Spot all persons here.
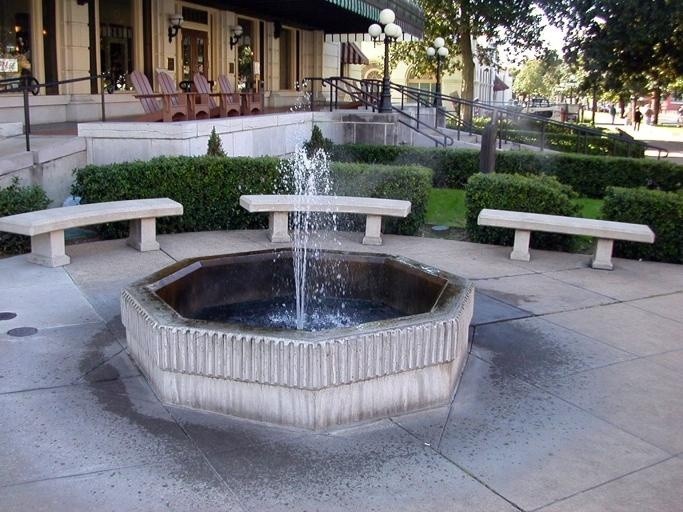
[633,105,643,131]
[610,104,616,124]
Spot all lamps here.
[165,12,186,44]
[228,24,245,50]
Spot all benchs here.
[0,196,188,270]
[475,205,658,272]
[236,192,414,247]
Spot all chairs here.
[337,79,383,110]
[124,67,265,123]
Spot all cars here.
[564,93,682,128]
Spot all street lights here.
[368,9,404,113]
[426,36,448,105]
